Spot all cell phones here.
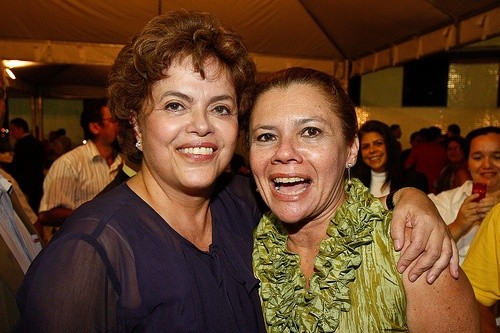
[471,181,487,204]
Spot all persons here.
[247,66,481,333]
[37,95,125,225]
[92,117,143,199]
[429,126,500,270]
[461,200,500,332]
[15,7,460,333]
[0,98,475,249]
